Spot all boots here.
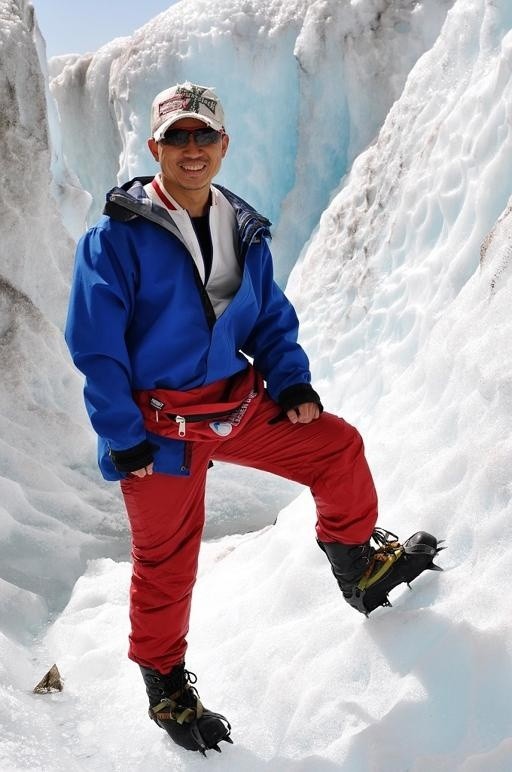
[140,663,233,754]
[315,530,441,615]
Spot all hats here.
[161,128,221,146]
[151,83,225,142]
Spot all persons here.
[62,78,437,756]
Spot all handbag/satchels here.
[141,365,264,442]
